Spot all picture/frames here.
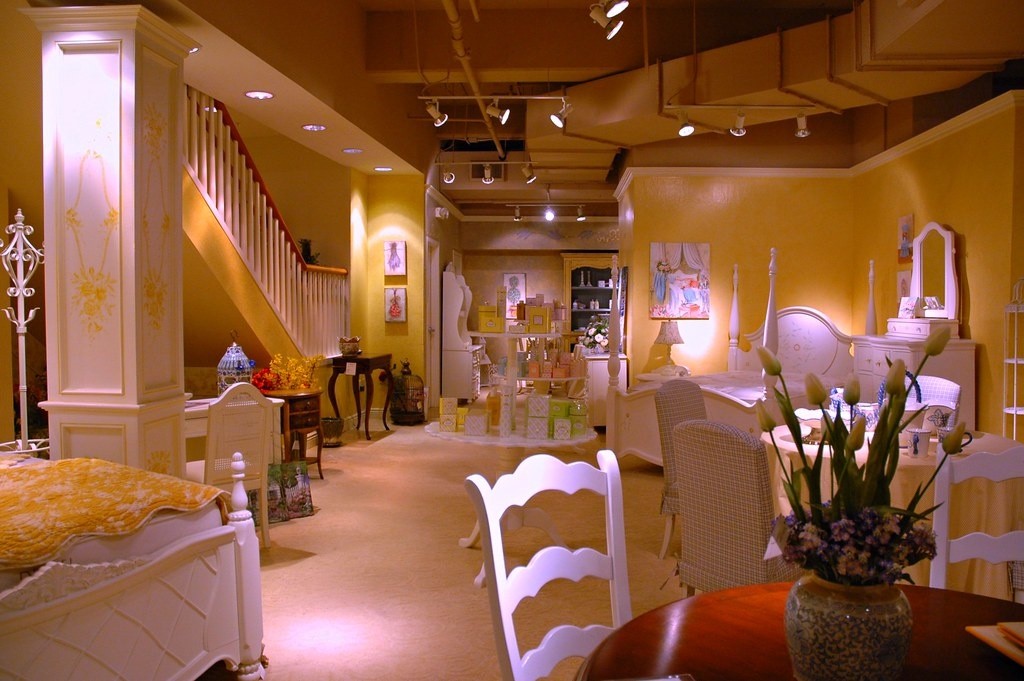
[383,241,407,276]
[384,287,407,322]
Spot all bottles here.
[590,299,595,310]
[573,300,578,309]
[517,300,525,319]
[595,299,599,309]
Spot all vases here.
[786,573,914,681]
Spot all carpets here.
[425,407,598,447]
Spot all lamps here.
[676,111,694,137]
[730,108,747,137]
[590,0,629,40]
[425,97,586,223]
[651,319,691,377]
[795,110,811,137]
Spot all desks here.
[574,582,1024,681]
[761,426,1024,601]
[465,330,563,431]
[329,354,395,441]
[185,397,285,466]
[634,372,689,382]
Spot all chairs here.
[465,375,1024,681]
[185,381,274,549]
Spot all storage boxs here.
[439,284,591,441]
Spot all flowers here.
[756,328,973,587]
[249,349,324,392]
[582,316,609,351]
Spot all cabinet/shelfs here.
[561,253,628,428]
[260,388,325,480]
[1002,278,1023,440]
[442,262,483,405]
[852,317,974,431]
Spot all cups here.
[598,280,605,288]
[936,427,973,448]
[830,392,883,430]
[907,428,932,459]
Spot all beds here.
[0,451,266,681]
[605,246,879,468]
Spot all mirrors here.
[909,222,960,319]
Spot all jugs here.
[897,402,929,448]
[922,399,956,436]
